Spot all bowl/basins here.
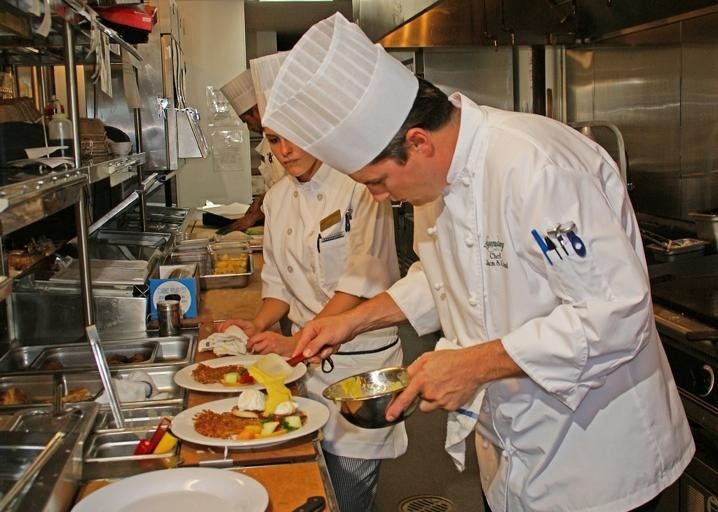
[109,142,132,154]
[322,365,420,429]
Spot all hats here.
[249,50,291,129]
[219,71,259,117]
[264,13,420,176]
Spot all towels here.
[436,338,485,472]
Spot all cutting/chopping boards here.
[150,253,283,329]
[74,461,331,512]
[179,322,318,466]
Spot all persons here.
[215,49,409,512]
[261,11,697,512]
[220,68,286,231]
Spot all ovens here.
[566,4,718,512]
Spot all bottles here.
[47,103,74,159]
[158,300,181,338]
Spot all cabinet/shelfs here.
[1,1,147,340]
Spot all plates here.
[116,205,193,241]
[29,340,159,372]
[164,238,253,253]
[173,355,307,392]
[0,334,194,373]
[71,467,269,512]
[85,430,178,462]
[0,375,105,407]
[170,396,330,449]
[91,228,172,257]
[148,252,254,289]
[93,406,183,433]
[248,235,264,250]
